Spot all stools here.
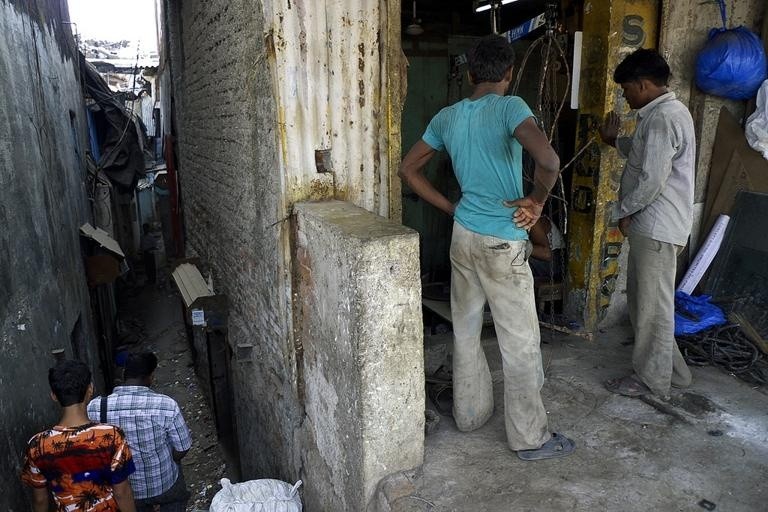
[532,275,562,313]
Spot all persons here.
[597,48,696,400]
[527,211,566,277]
[20,359,139,511]
[399,34,574,461]
[87,349,192,511]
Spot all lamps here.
[472,0,519,13]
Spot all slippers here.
[604,375,651,398]
[516,433,575,461]
